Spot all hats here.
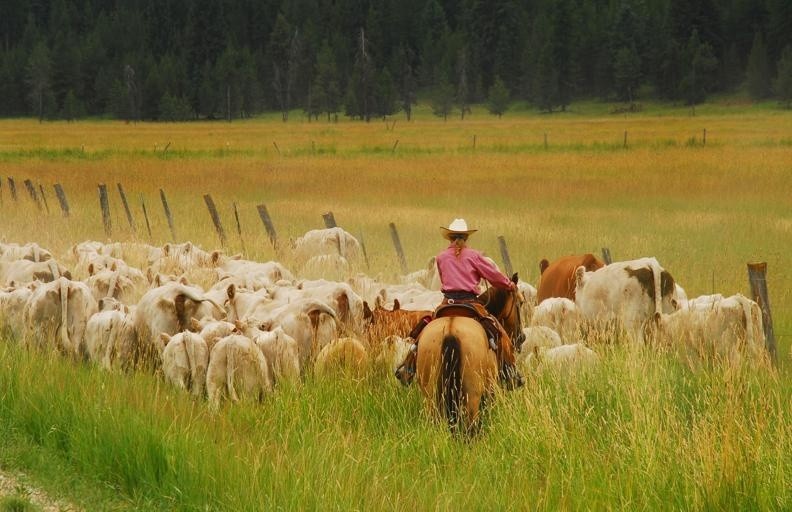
[440,218,478,240]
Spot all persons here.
[395,218,527,392]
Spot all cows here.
[0,227,445,417]
[479,253,765,379]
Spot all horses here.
[414,272,526,444]
